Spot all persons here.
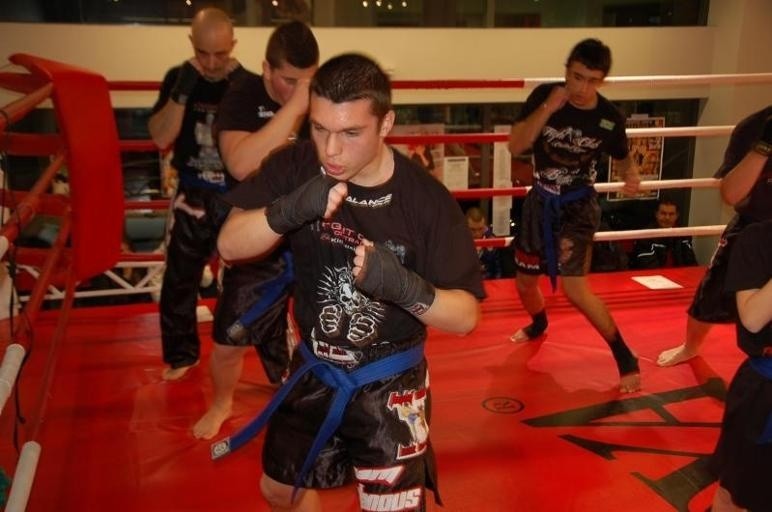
[190,14,322,441]
[412,146,436,170]
[630,195,699,271]
[211,52,490,512]
[147,6,291,391]
[112,240,143,291]
[465,204,502,279]
[703,217,770,511]
[507,35,642,396]
[654,105,772,367]
[589,214,623,273]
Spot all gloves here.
[353,242,437,318]
[752,115,771,157]
[265,174,340,237]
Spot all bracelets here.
[540,100,554,117]
